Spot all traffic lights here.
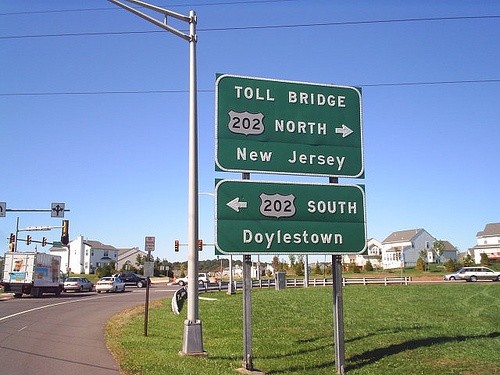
[197,240,202,251]
[175,240,179,252]
[42,237,46,246]
[9,233,15,243]
[61,219,70,239]
[27,234,31,245]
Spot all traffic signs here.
[51,202,64,217]
[214,73,365,179]
[0,202,6,217]
[214,178,368,257]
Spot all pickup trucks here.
[176,272,221,286]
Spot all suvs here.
[114,273,150,288]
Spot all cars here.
[63,277,93,293]
[96,277,125,293]
[460,268,500,282]
[444,267,466,280]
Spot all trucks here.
[2,251,64,297]
